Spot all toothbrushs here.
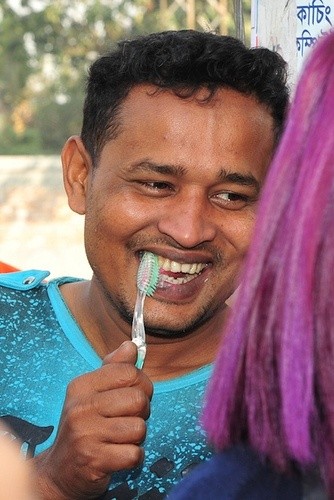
[131,252,160,370]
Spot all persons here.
[0,30,288,500]
[166,29,334,500]
